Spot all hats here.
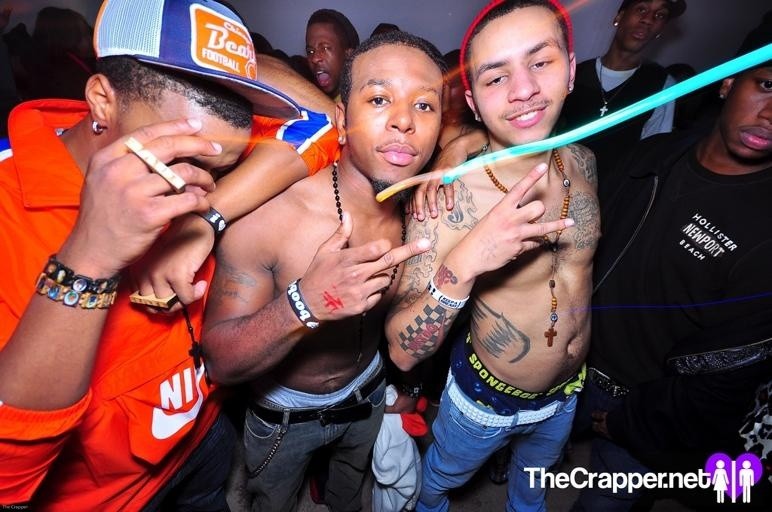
[618,0,686,21]
[91,0,301,120]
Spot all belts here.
[445,366,567,427]
[250,366,386,426]
